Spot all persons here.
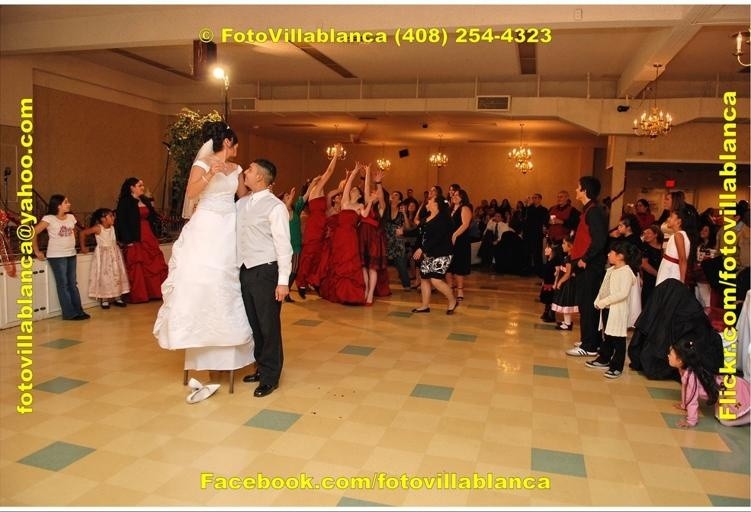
[153,121,257,374]
[31,193,91,321]
[233,157,293,397]
[80,208,131,310]
[115,177,168,304]
[277,140,751,430]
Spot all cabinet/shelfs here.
[0,260,39,329]
[39,254,101,322]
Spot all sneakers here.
[540,311,623,378]
[113,301,127,307]
[73,313,91,320]
[101,300,110,309]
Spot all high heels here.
[186,377,222,404]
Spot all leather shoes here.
[242,369,262,382]
[283,274,464,315]
[254,382,279,397]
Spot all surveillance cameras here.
[617,105,629,112]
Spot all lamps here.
[633,64,672,137]
[428,133,448,169]
[326,123,347,161]
[506,123,531,162]
[736,27,751,70]
[376,143,391,172]
[516,163,534,175]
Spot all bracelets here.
[202,175,210,184]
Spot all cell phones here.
[550,215,556,224]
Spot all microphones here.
[5,166,11,175]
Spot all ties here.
[495,224,498,239]
